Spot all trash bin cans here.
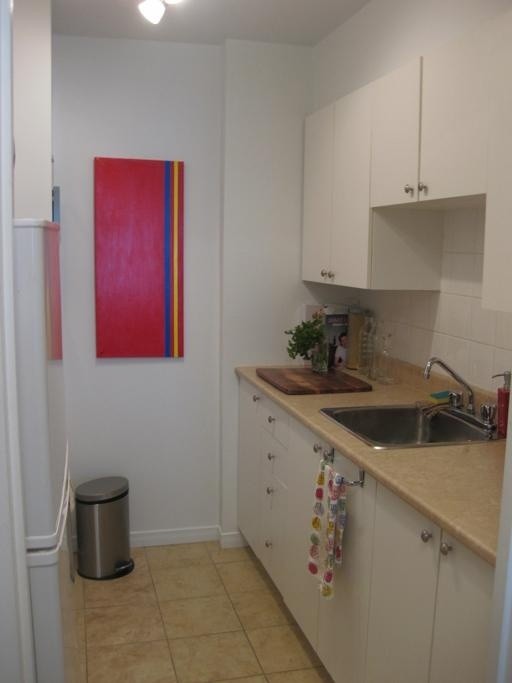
[74,474,133,581]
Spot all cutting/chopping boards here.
[256,367,372,394]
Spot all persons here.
[334,332,348,367]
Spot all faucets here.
[423,357,475,414]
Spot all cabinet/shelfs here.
[479,10,510,304]
[371,31,485,212]
[237,378,290,598]
[365,437,498,682]
[302,86,443,293]
[282,418,376,680]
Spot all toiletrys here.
[490,371,512,438]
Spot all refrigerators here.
[13,219,73,683]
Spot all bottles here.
[356,316,399,384]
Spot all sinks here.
[318,402,506,450]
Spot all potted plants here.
[285,312,329,374]
